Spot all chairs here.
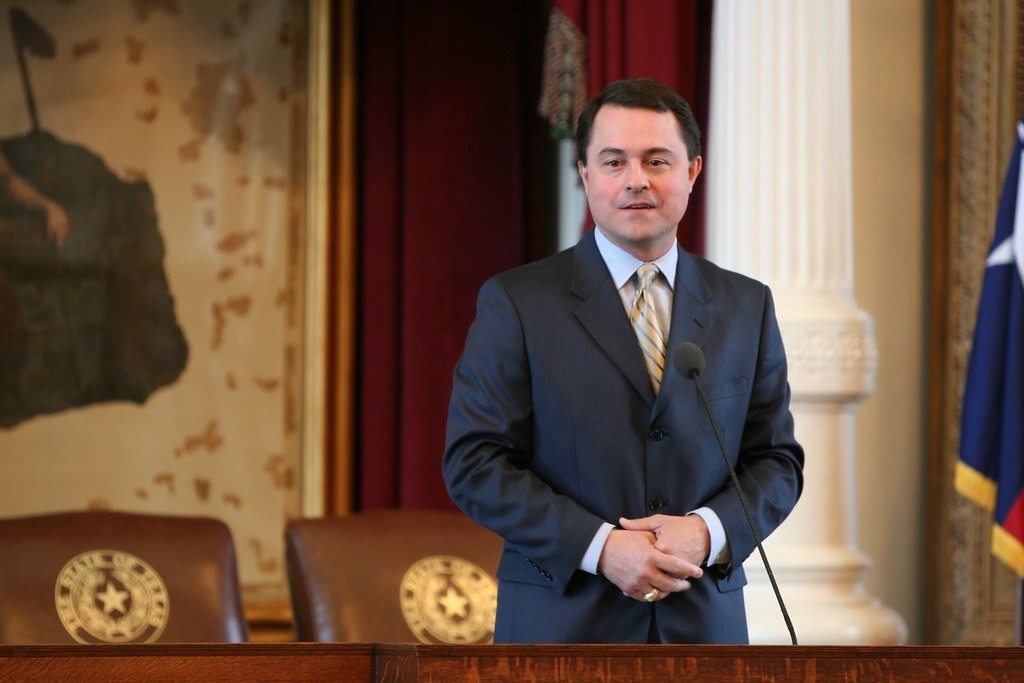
[0,509,248,645]
[283,512,505,645]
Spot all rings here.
[643,589,658,603]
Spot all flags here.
[954,116,1024,578]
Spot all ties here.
[631,264,668,395]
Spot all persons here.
[443,78,804,644]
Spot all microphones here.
[673,342,801,645]
